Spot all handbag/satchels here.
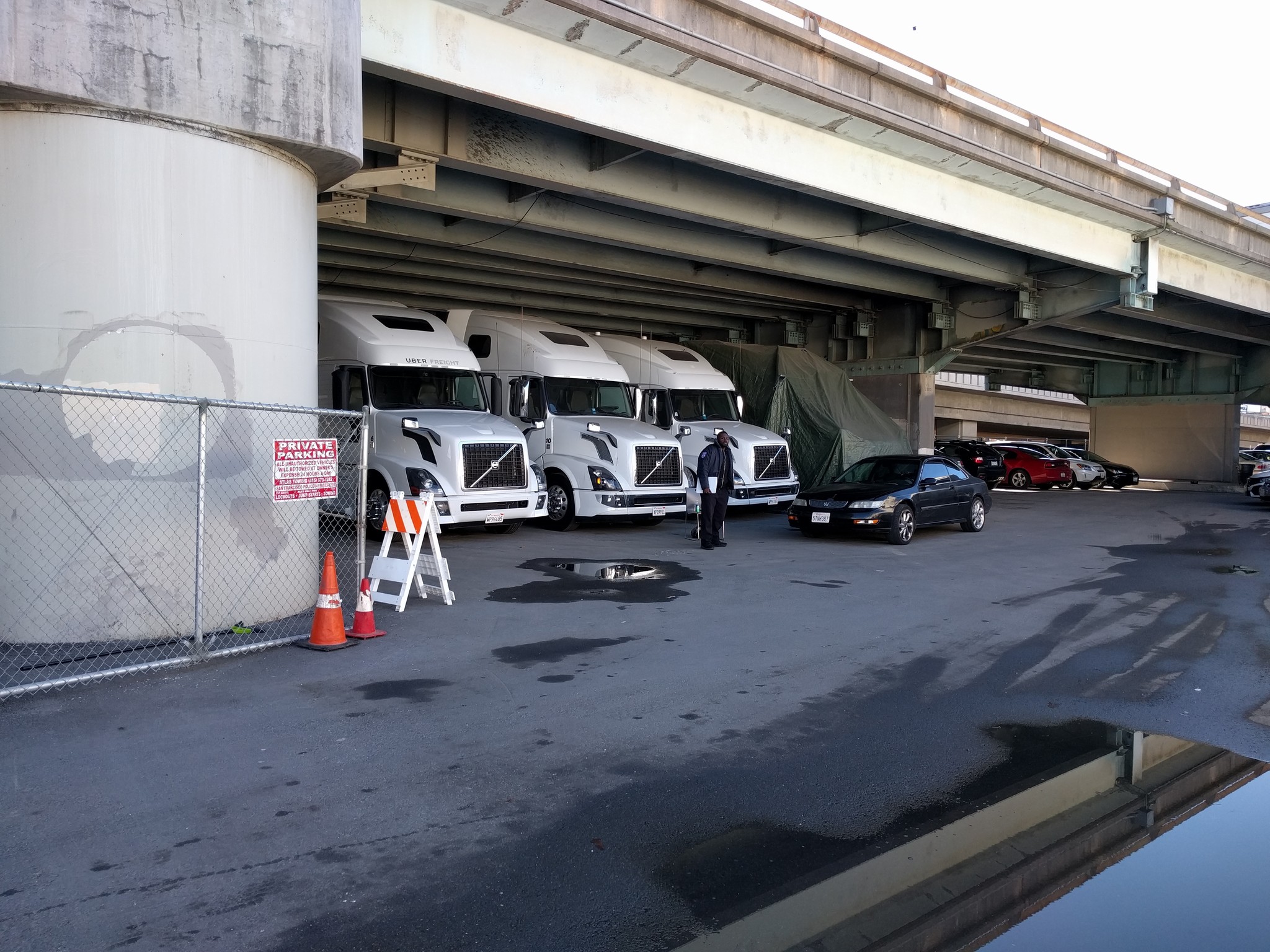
[691,523,701,538]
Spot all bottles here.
[693,500,699,511]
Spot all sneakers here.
[712,540,727,547]
[701,543,714,549]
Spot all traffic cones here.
[346,578,388,641]
[294,551,362,652]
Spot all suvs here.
[934,439,1008,491]
[1253,443,1270,451]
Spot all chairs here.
[677,399,698,418]
[418,382,439,405]
[567,391,591,412]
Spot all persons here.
[697,431,734,549]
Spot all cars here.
[1060,447,1140,489]
[985,441,1107,491]
[987,446,1073,489]
[1244,470,1270,502]
[1238,452,1270,474]
[932,448,965,471]
[1239,450,1270,461]
[786,455,996,544]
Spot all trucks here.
[583,331,802,513]
[318,296,550,536]
[433,310,691,531]
